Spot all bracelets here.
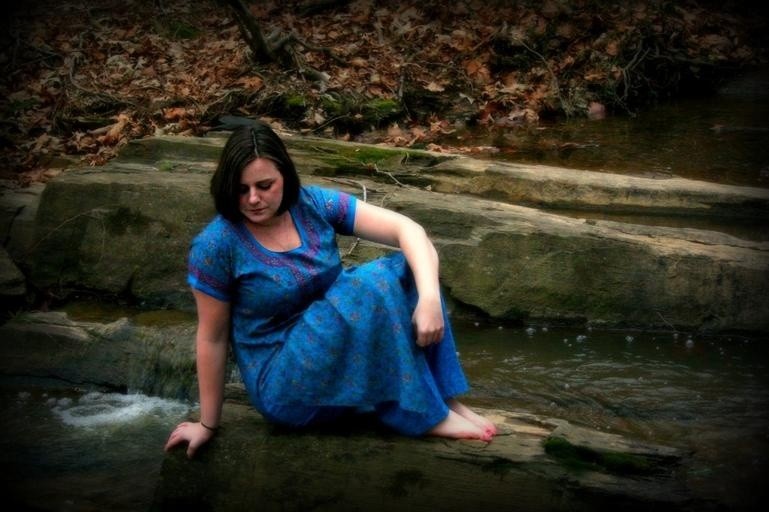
[201,420,220,431]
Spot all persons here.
[165,124,497,459]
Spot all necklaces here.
[262,215,288,253]
